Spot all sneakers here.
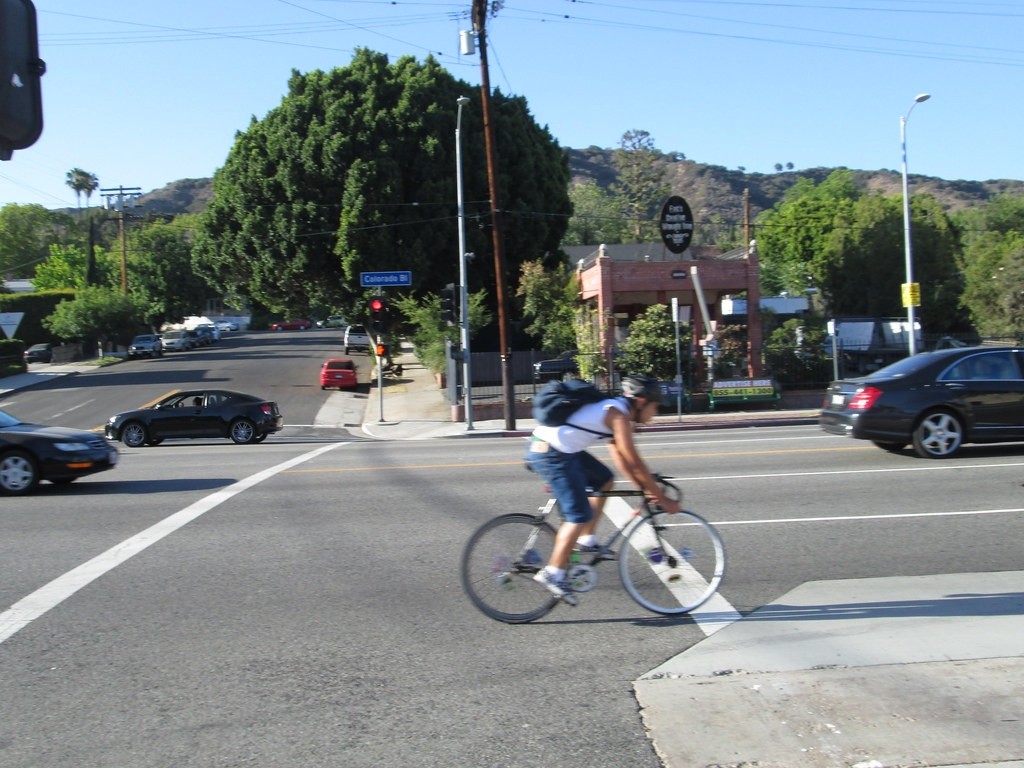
[573,542,619,561]
[533,568,578,605]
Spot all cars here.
[0,411,119,498]
[105,389,282,448]
[320,359,359,392]
[129,316,239,358]
[23,344,54,364]
[817,346,1024,460]
[535,349,579,381]
[271,319,311,331]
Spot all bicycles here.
[460,472,726,625]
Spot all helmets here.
[623,371,664,402]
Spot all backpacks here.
[532,378,630,438]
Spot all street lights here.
[455,93,478,436]
[898,93,933,357]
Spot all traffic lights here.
[376,343,390,356]
[368,295,387,315]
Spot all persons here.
[523,373,680,607]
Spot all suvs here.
[344,324,370,354]
[317,315,349,328]
